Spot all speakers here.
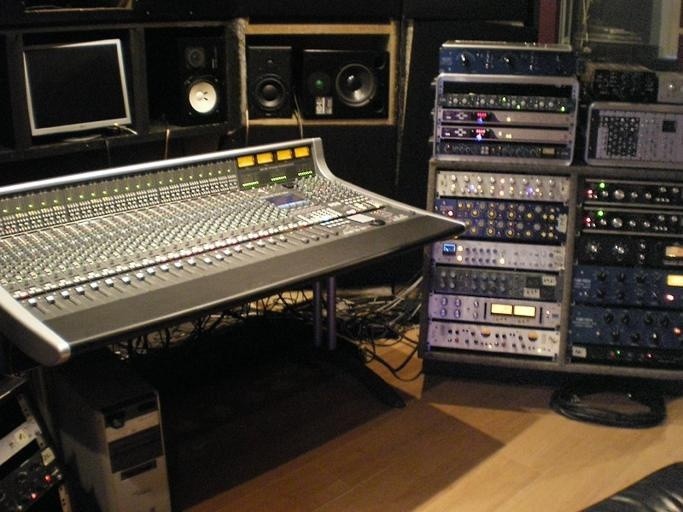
[148,26,228,127]
[302,49,389,119]
[246,45,293,119]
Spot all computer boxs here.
[44,347,172,512]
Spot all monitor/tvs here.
[22,38,133,142]
